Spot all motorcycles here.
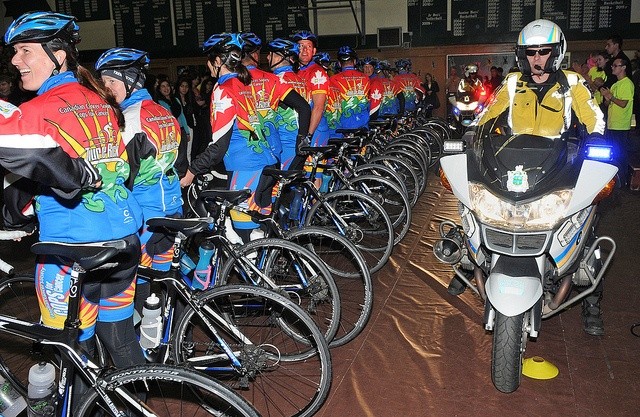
[448,89,486,134]
[433,74,619,392]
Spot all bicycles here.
[297,144,412,253]
[180,188,340,361]
[307,137,411,252]
[0,238,263,416]
[180,168,373,348]
[0,216,332,416]
[335,126,419,219]
[279,144,394,277]
[369,102,452,169]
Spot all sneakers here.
[581,296,604,336]
[448,265,475,296]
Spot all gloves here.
[296,136,309,156]
[79,158,102,187]
[461,126,480,147]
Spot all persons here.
[0,77,13,102]
[483,76,491,92]
[192,74,214,155]
[267,38,308,171]
[143,74,159,103]
[95,46,184,339]
[490,66,503,88]
[8,73,38,107]
[600,57,634,189]
[594,37,632,118]
[294,30,330,205]
[448,66,460,93]
[154,80,191,179]
[361,57,383,120]
[586,59,592,69]
[379,58,426,116]
[477,76,482,83]
[240,33,311,169]
[589,50,599,66]
[448,19,606,335]
[422,73,440,117]
[498,67,505,79]
[0,11,144,417]
[456,63,483,102]
[585,52,611,104]
[157,73,169,84]
[326,46,371,164]
[179,32,277,241]
[174,78,197,167]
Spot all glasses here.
[612,63,624,68]
[526,48,553,56]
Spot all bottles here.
[27,362,59,417]
[319,169,333,193]
[245,228,265,275]
[139,292,163,350]
[289,182,304,219]
[192,239,215,291]
[350,156,357,167]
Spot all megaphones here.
[433,228,464,264]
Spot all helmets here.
[515,19,567,76]
[337,45,358,60]
[395,59,410,67]
[4,11,81,47]
[375,60,391,70]
[292,31,318,48]
[241,33,262,46]
[361,57,377,66]
[200,32,247,52]
[464,65,479,78]
[265,38,300,56]
[95,48,151,72]
[312,52,331,67]
[177,67,189,76]
